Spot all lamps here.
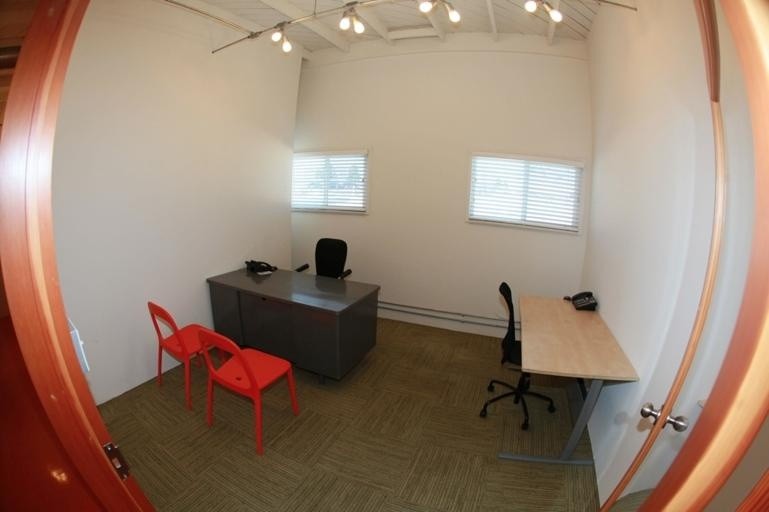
[210,0,638,55]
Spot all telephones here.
[569,291,597,311]
[246,260,277,272]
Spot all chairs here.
[148,302,225,412]
[294,237,352,280]
[480,281,556,431]
[199,329,300,455]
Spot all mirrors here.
[1,0,768,510]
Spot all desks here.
[207,265,381,385]
[498,294,639,465]
